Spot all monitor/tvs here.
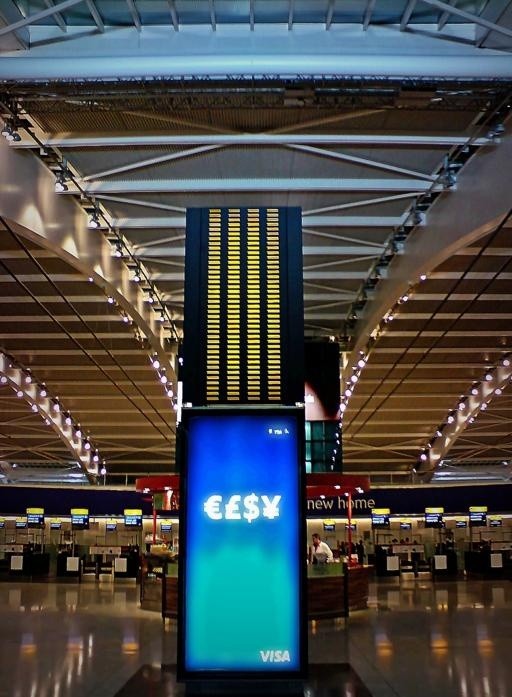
[372,515,390,529]
[425,514,442,528]
[16,522,26,529]
[71,514,90,530]
[324,525,335,532]
[456,522,466,528]
[106,524,117,532]
[469,513,486,527]
[0,522,5,528]
[26,514,44,528]
[489,520,501,527]
[400,523,411,530]
[50,523,61,530]
[345,525,356,531]
[161,525,172,532]
[124,516,143,528]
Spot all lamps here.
[2,125,10,137]
[88,243,180,412]
[6,131,15,141]
[1,363,106,481]
[421,356,511,466]
[55,177,65,192]
[88,215,99,229]
[379,123,507,268]
[330,273,427,469]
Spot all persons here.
[388,537,420,565]
[336,539,375,565]
[309,532,334,563]
